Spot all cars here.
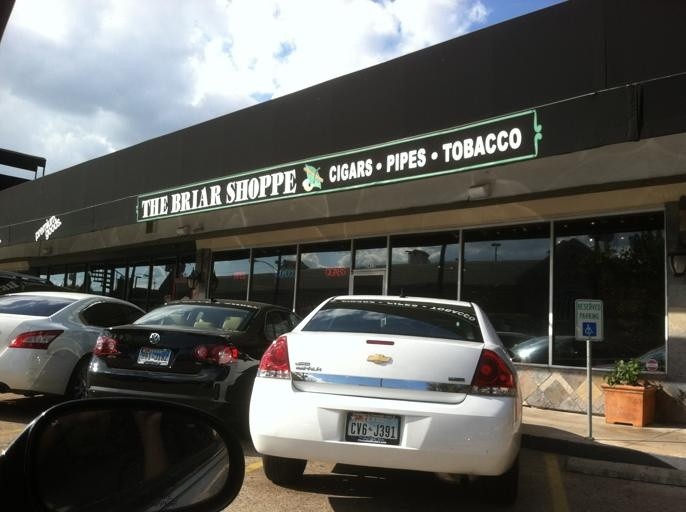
[497,331,668,372]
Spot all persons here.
[38,408,169,511]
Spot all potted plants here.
[602,360,659,425]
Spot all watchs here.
[51,419,65,440]
